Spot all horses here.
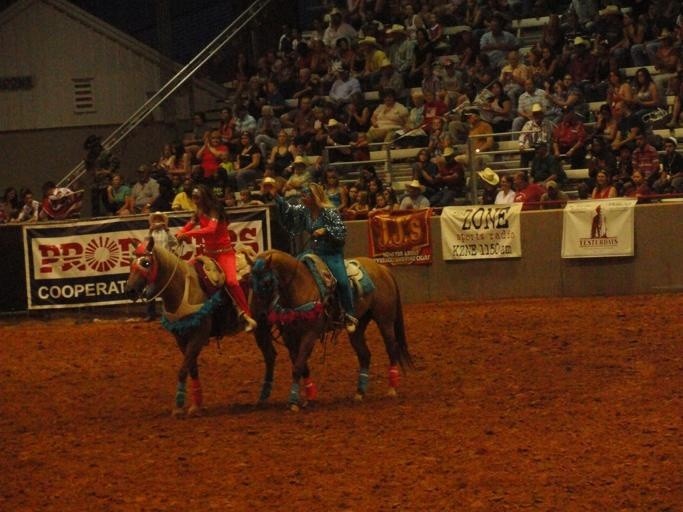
[124,237,277,420]
[240,249,416,413]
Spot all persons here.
[174,184,258,333]
[262,183,357,334]
[83,136,118,217]
[0,181,75,224]
[106,0,683,225]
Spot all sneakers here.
[245,321,254,332]
[345,317,356,333]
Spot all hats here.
[329,7,405,72]
[599,4,619,15]
[573,36,591,49]
[658,28,672,40]
[545,180,558,190]
[463,106,479,114]
[532,104,547,114]
[405,148,500,193]
[258,118,339,191]
[666,136,678,147]
